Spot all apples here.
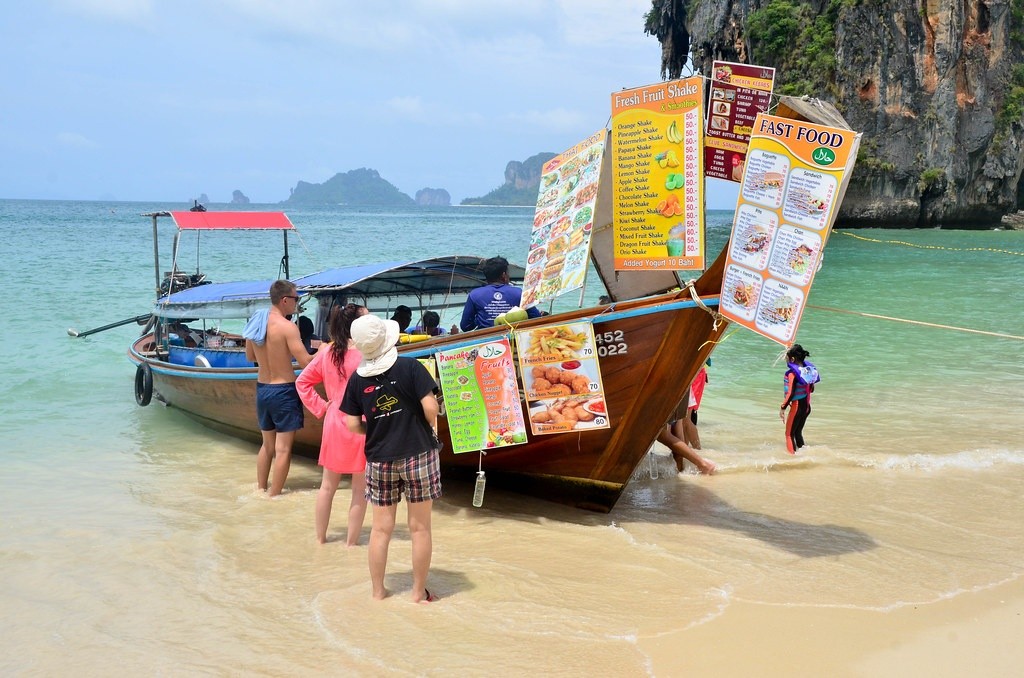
[513,431,526,442]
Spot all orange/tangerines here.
[665,173,684,189]
[656,193,683,217]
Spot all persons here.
[681,349,713,451]
[254,314,292,367]
[245,280,328,496]
[388,305,413,335]
[658,387,716,475]
[460,255,541,334]
[293,316,321,359]
[338,313,439,603]
[294,303,377,548]
[779,343,821,455]
[410,310,445,338]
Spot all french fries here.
[527,325,589,362]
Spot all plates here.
[530,405,607,430]
[582,399,606,417]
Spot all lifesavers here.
[135,362,153,406]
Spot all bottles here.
[472,471,486,508]
[649,450,658,480]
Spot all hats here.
[351,315,400,377]
[390,304,412,321]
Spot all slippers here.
[424,588,433,601]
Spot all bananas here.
[666,120,684,143]
[488,429,499,442]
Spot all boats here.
[65,93,854,516]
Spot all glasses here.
[396,316,412,322]
[280,295,299,302]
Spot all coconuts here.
[494,305,528,325]
